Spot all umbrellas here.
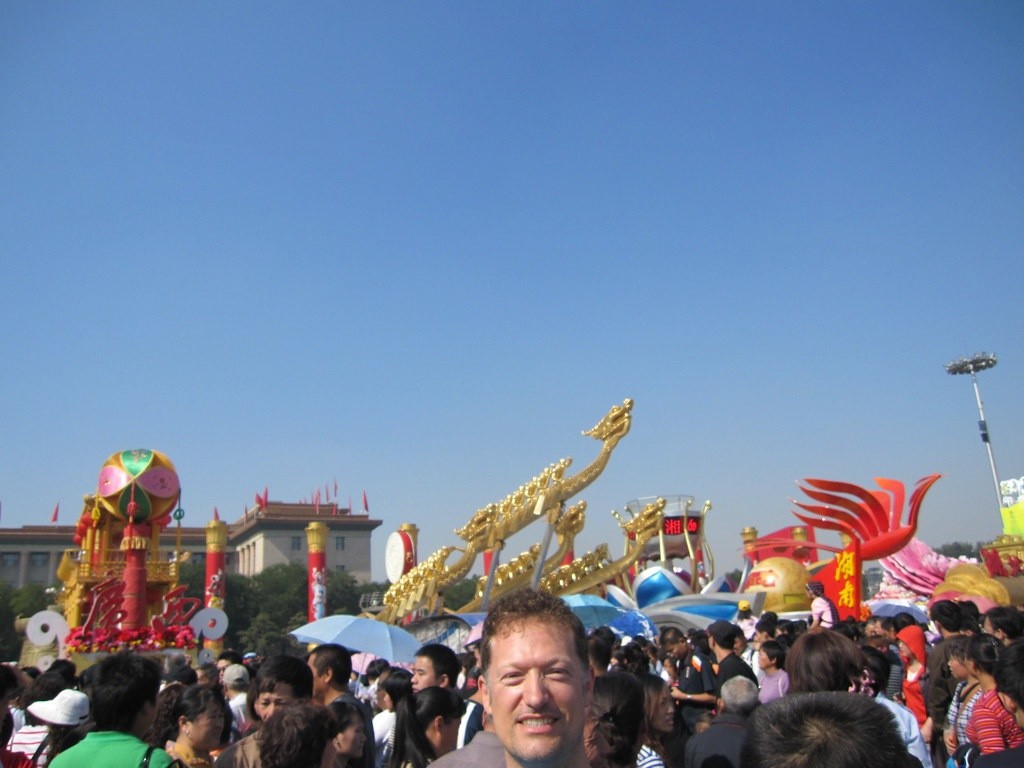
[561,594,628,628]
[289,615,424,696]
[607,611,657,637]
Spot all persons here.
[478,587,595,768]
[0,642,506,768]
[584,582,1024,768]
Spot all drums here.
[385,531,415,584]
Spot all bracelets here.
[687,695,691,702]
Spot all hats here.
[222,663,250,686]
[26,687,90,726]
[737,599,750,611]
[162,666,198,686]
[706,620,737,644]
